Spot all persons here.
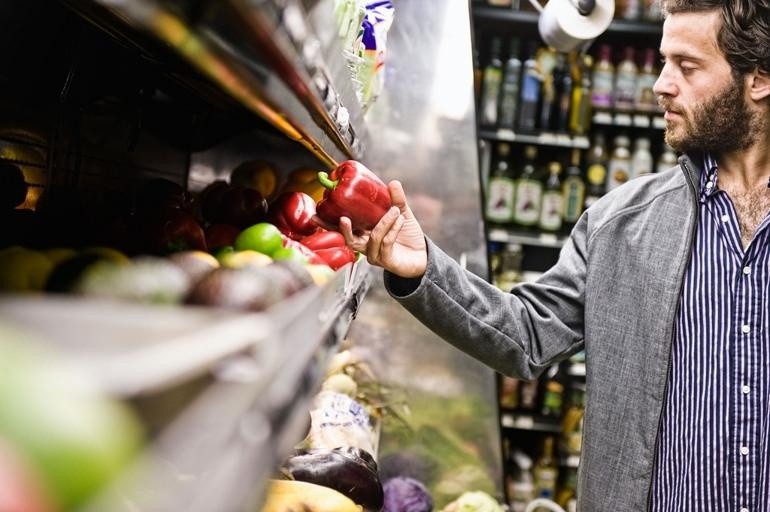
[333,1,770,512]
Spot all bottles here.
[487,239,558,293]
[471,30,663,136]
[502,434,578,512]
[498,357,585,425]
[485,133,679,236]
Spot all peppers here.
[138,159,394,272]
